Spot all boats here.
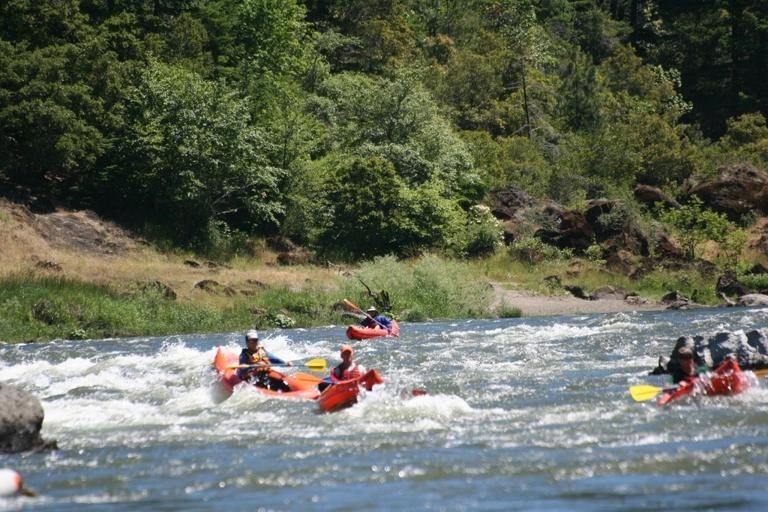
[345,317,399,340]
[658,371,758,407]
[212,348,429,415]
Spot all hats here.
[341,345,353,355]
[246,332,258,341]
[367,306,378,314]
[678,347,692,355]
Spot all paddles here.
[628,377,697,401]
[343,299,399,337]
[294,373,426,396]
[225,357,327,372]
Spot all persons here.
[673,348,705,383]
[359,306,392,332]
[318,346,367,392]
[239,332,293,391]
